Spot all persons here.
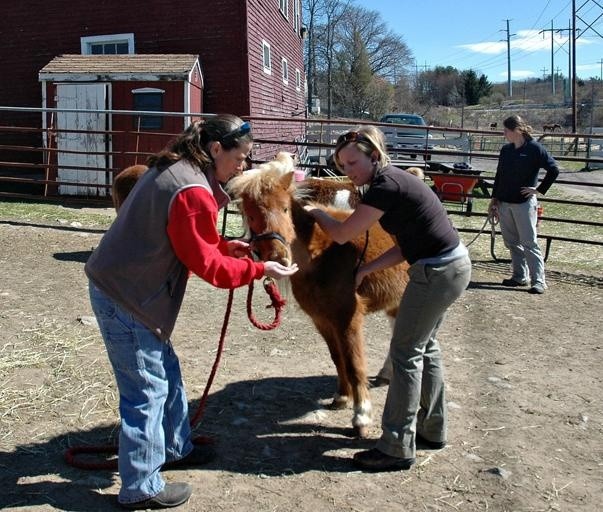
[82,112,300,510]
[302,125,472,471]
[487,113,560,294]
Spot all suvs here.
[376,115,433,161]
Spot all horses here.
[268,151,425,211]
[543,124,561,133]
[112,165,150,216]
[491,123,497,130]
[223,164,416,439]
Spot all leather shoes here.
[125,482,191,509]
[158,442,211,469]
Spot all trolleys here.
[420,169,482,218]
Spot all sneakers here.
[530,283,544,294]
[354,449,417,472]
[416,433,443,449]
[503,278,528,286]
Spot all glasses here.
[217,122,250,141]
[335,132,370,147]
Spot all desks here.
[424,158,496,200]
